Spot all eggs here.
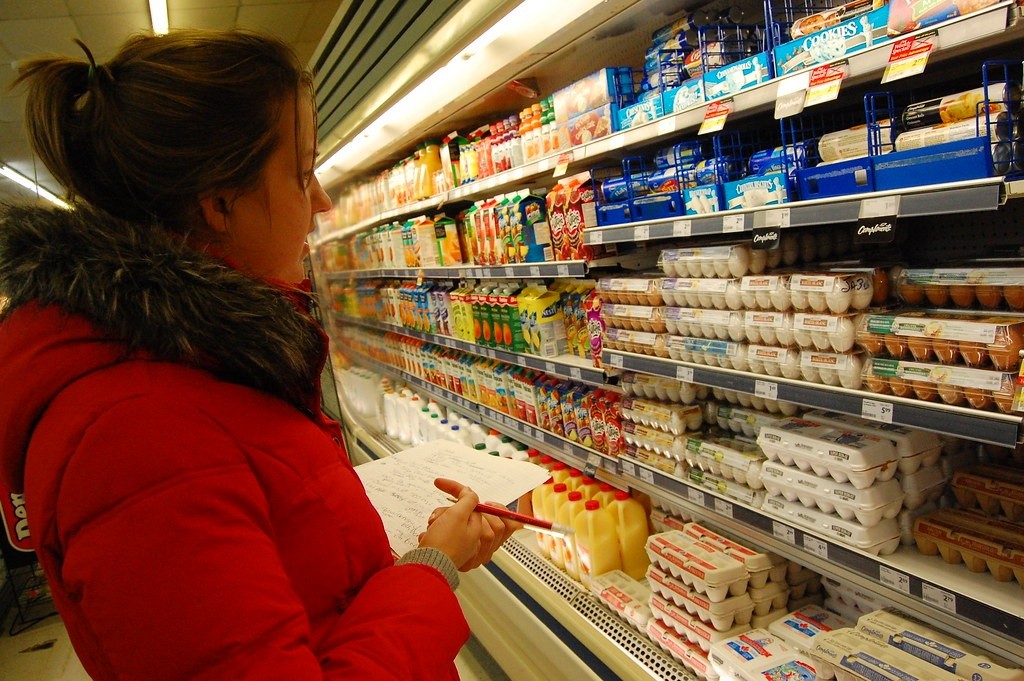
[597,233,1024,488]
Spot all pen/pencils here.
[446,497,576,538]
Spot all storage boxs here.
[554,5,895,153]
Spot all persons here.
[0,26,523,681]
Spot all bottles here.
[308,97,558,240]
[329,362,651,594]
